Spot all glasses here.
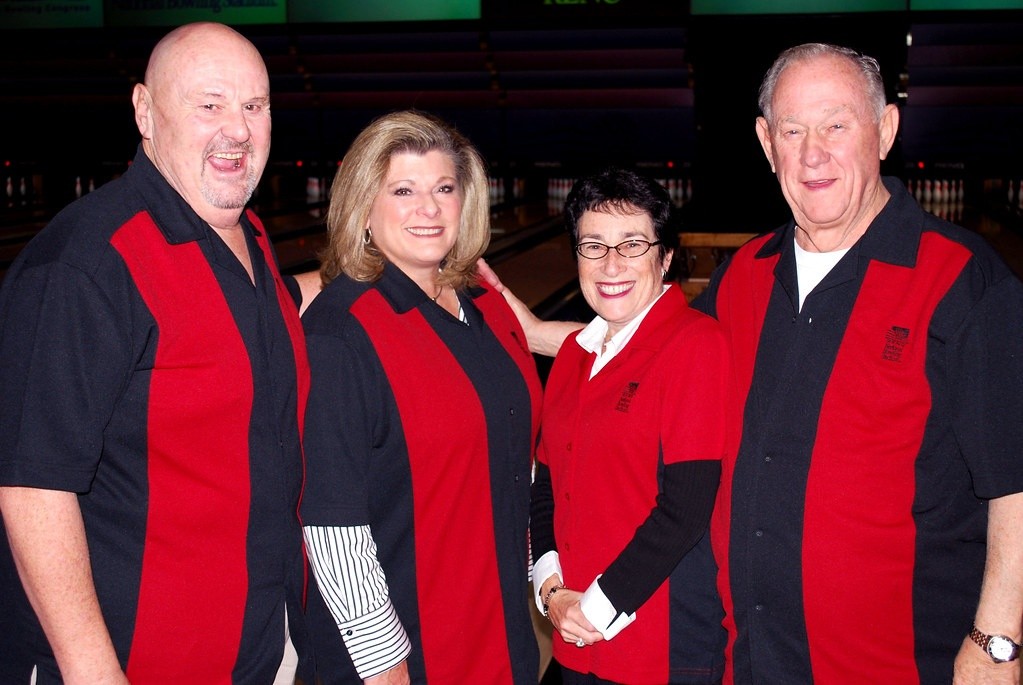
[574,239,661,258]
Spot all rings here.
[576,638,585,647]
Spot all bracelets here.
[544,584,568,620]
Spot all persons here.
[477,42,1023,685]
[286,112,544,685]
[528,166,730,685]
[0,19,342,685]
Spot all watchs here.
[967,623,1022,664]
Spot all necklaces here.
[430,286,444,302]
